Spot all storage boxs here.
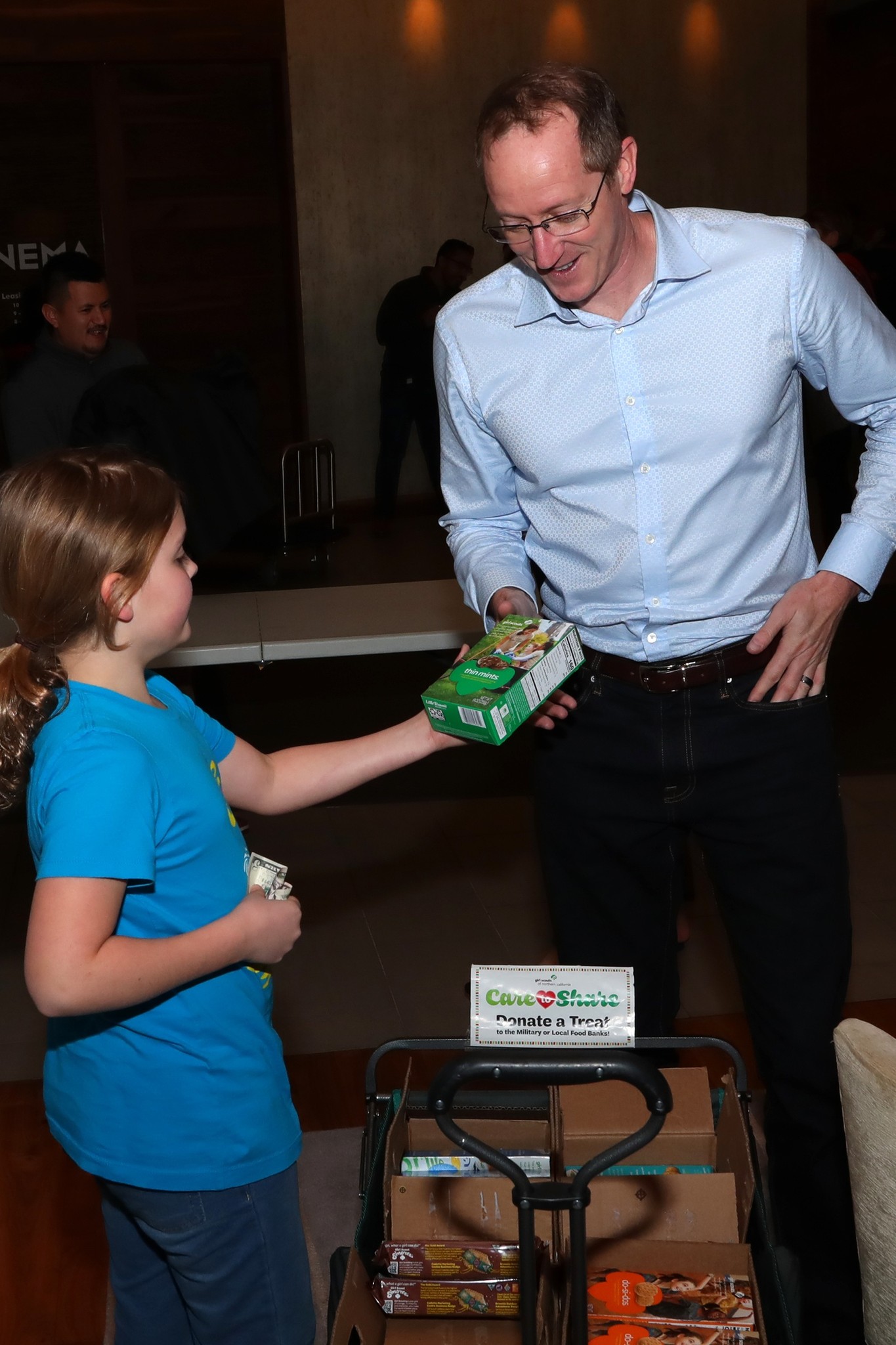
[421,609,587,748]
[383,1053,555,1247]
[561,1235,769,1345]
[326,1238,558,1345]
[558,1065,759,1257]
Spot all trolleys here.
[327,1034,795,1345]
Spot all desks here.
[145,576,489,671]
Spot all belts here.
[582,633,781,693]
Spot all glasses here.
[481,160,611,244]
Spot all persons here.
[0,439,580,1345]
[428,68,896,1344]
[372,240,476,537]
[1,251,165,466]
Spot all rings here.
[801,675,813,687]
